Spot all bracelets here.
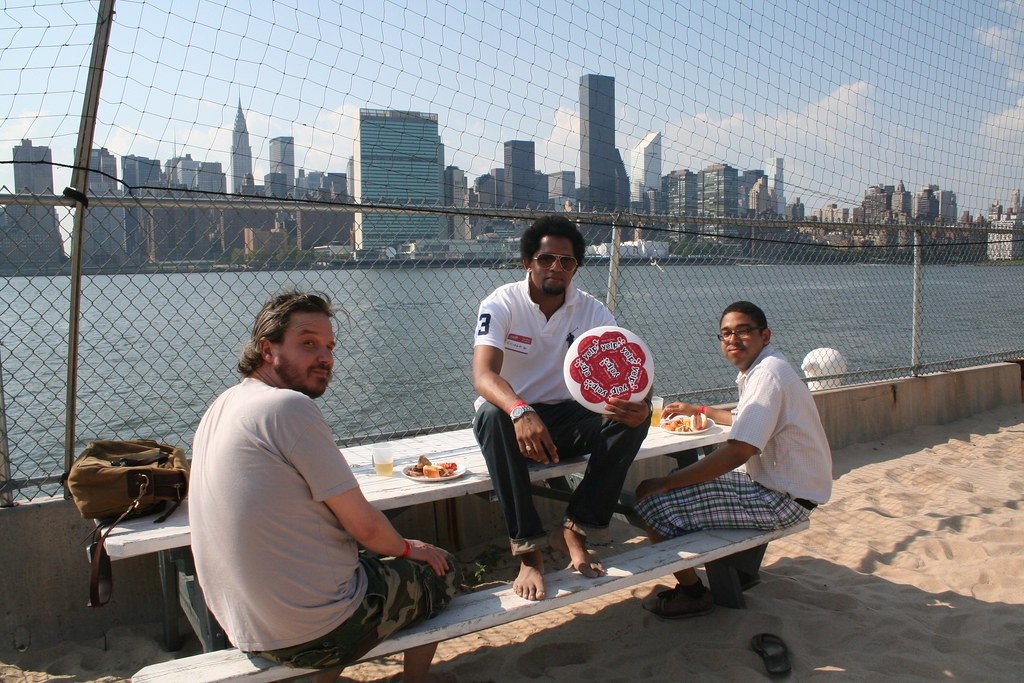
[400,540,411,558]
[644,397,653,415]
[507,401,527,415]
[700,406,706,414]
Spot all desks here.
[94,408,733,650]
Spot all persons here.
[634,301,833,618]
[472,214,653,601]
[187,292,463,683]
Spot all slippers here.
[751,633,791,674]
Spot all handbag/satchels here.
[68,438,190,520]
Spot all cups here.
[371,443,394,476]
[650,396,664,427]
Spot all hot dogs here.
[690,412,707,431]
[423,464,448,478]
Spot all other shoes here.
[643,586,715,619]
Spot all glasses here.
[718,326,763,341]
[533,253,578,272]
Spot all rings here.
[526,446,534,451]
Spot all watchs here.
[510,406,536,423]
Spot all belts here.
[794,497,817,510]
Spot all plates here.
[401,461,467,482]
[659,417,716,435]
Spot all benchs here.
[131,517,813,683]
